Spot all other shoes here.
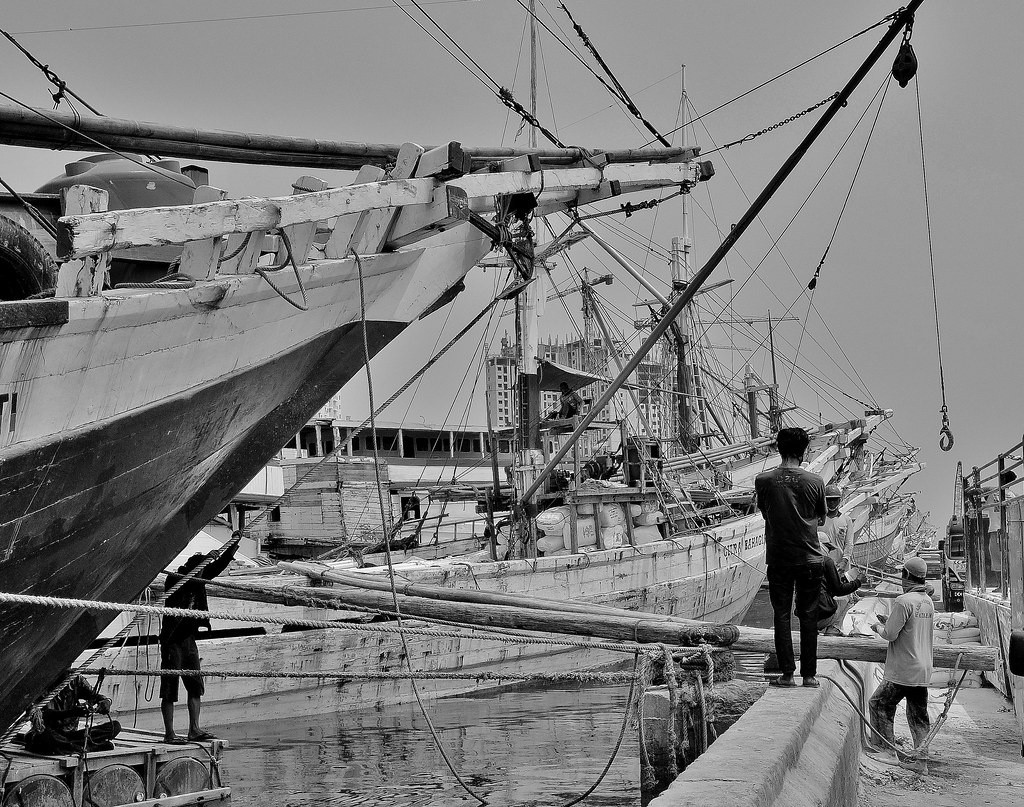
[770,676,795,687]
[803,676,820,686]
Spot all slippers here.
[187,732,219,741]
[164,735,187,741]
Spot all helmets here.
[825,485,842,497]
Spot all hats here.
[817,532,839,548]
[904,557,928,578]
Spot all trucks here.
[917,551,943,580]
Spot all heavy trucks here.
[938,514,976,613]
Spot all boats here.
[0,0,713,750]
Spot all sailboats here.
[73,0,927,711]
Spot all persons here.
[817,484,854,576]
[23,667,121,754]
[793,531,867,636]
[968,470,1017,593]
[755,427,828,686]
[159,529,243,744]
[869,557,933,775]
[544,380,585,420]
